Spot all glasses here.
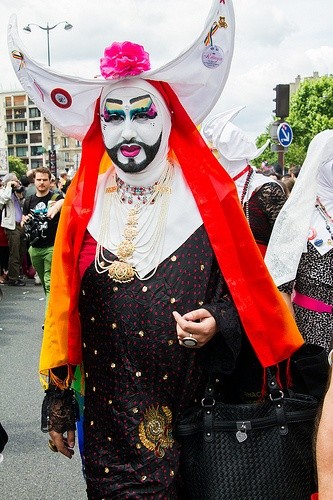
[36,178,48,181]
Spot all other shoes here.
[7,277,25,286]
[34,275,42,285]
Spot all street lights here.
[24,20,74,179]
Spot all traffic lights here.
[271,84,290,116]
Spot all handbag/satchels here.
[173,349,318,500]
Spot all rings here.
[48,440,57,453]
[182,332,198,347]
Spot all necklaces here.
[240,168,253,205]
[246,170,256,226]
[315,195,333,241]
[94,160,174,283]
[113,175,160,207]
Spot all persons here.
[5,1,316,500]
[0,106,333,500]
